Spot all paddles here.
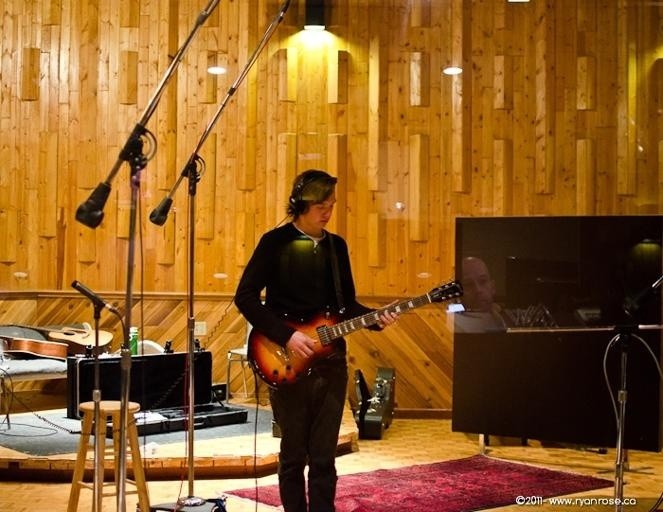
[221,451,626,512]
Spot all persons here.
[235,170,403,512]
[459,256,494,312]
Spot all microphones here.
[67,400,151,512]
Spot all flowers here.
[72,280,112,311]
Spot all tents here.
[286,170,328,215]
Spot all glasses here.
[302,0,326,28]
